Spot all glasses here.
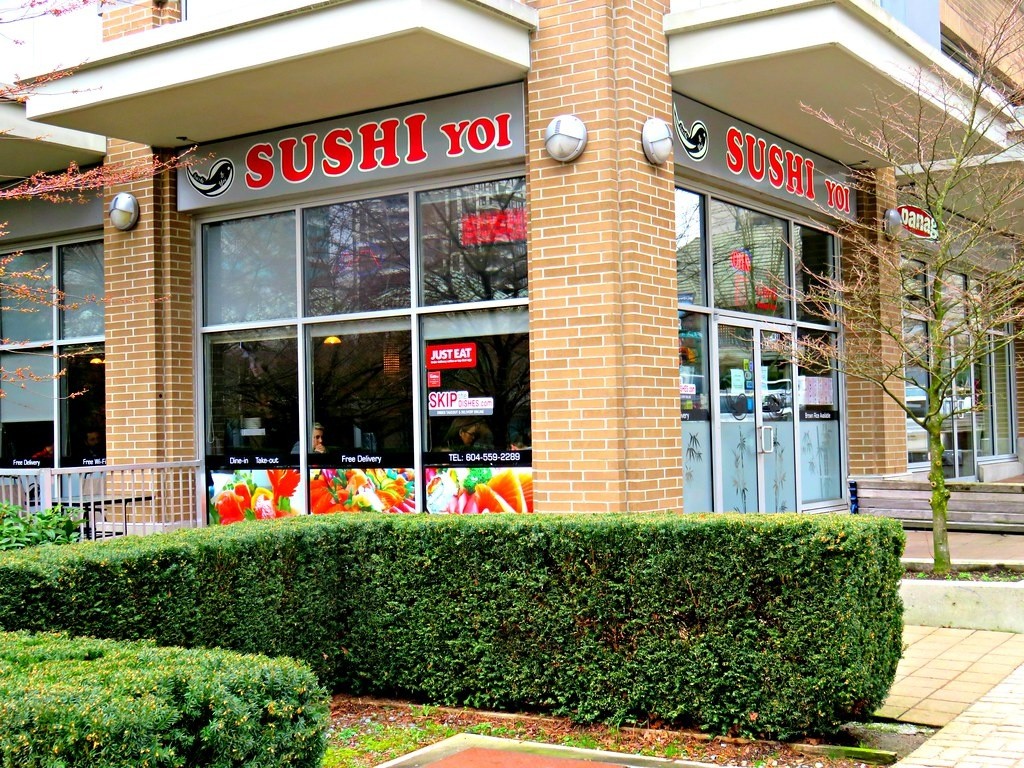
[464,431,476,437]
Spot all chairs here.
[0,472,106,541]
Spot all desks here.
[25,496,153,539]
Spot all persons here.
[291,422,330,454]
[74,427,106,466]
[242,349,287,467]
[442,414,532,450]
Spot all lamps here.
[883,209,903,242]
[543,114,587,162]
[640,118,673,165]
[108,192,139,232]
